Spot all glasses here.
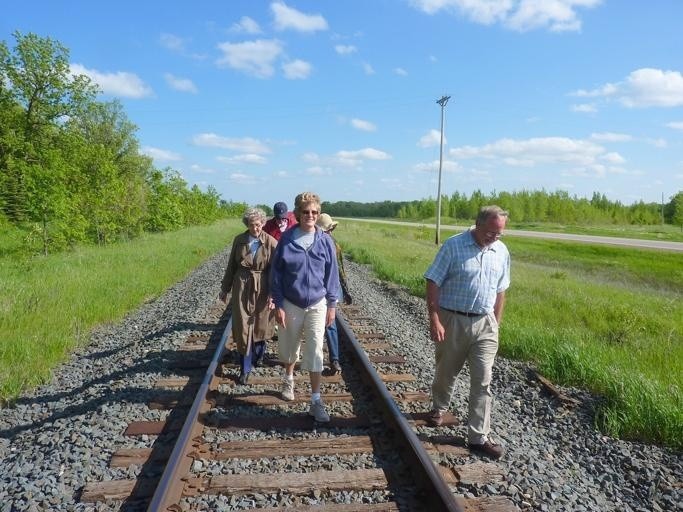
[302,210,318,214]
[486,231,501,238]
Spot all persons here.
[262,202,297,240]
[316,213,352,375]
[271,192,341,423]
[219,207,278,385]
[423,205,511,457]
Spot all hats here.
[273,202,288,218]
[315,213,338,232]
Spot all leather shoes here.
[239,373,248,385]
[479,440,503,456]
[430,410,443,426]
[331,360,341,371]
[256,358,263,366]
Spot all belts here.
[440,306,482,317]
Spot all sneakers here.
[281,378,295,400]
[309,401,330,422]
